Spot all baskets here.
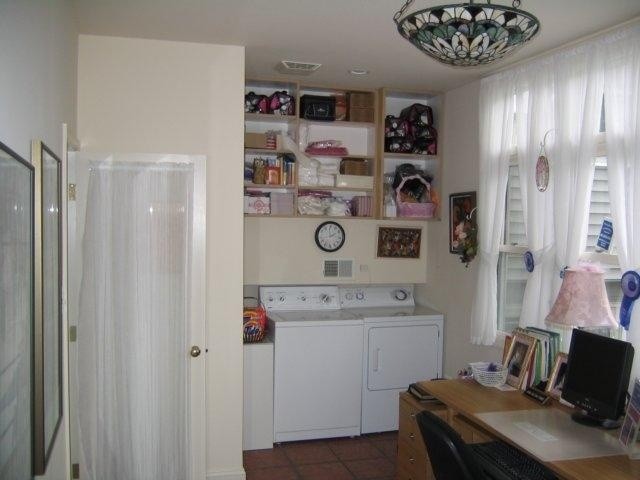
[395,173,434,217]
[469,360,509,388]
[346,91,375,122]
[243,297,266,343]
[341,160,368,176]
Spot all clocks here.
[315,223,344,251]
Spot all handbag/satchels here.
[300,94,335,121]
[244,91,295,115]
[385,103,437,155]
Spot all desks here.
[398,375,639,480]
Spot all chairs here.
[419,410,485,480]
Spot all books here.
[410,383,435,399]
[501,326,561,393]
[406,391,444,405]
[353,197,372,216]
[276,155,296,185]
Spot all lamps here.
[394,0,540,68]
[546,268,617,333]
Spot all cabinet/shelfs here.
[245,76,443,222]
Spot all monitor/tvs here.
[559,328,634,429]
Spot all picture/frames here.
[503,326,575,408]
[449,190,480,255]
[372,226,423,259]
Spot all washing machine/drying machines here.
[259,285,365,446]
[340,287,445,435]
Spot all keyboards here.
[465,440,559,480]
